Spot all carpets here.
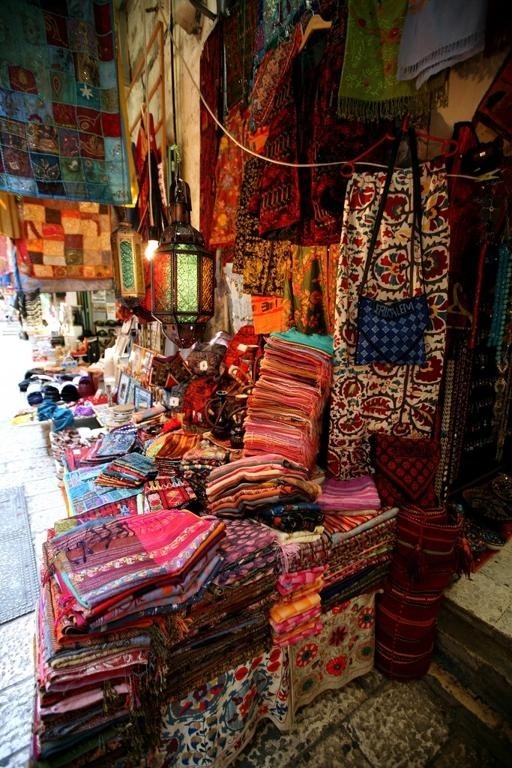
[0,483,42,625]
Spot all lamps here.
[142,0,160,262]
[152,0,215,346]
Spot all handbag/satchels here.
[147,351,193,387]
[368,433,441,508]
[355,294,428,365]
